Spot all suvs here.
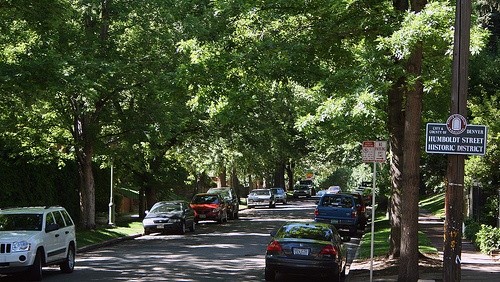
[0,205,78,282]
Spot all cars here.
[189,192,228,223]
[246,189,276,208]
[293,185,311,198]
[264,222,348,282]
[337,191,366,220]
[141,200,196,235]
[270,188,287,205]
[327,185,341,195]
[315,193,358,229]
[206,186,238,219]
